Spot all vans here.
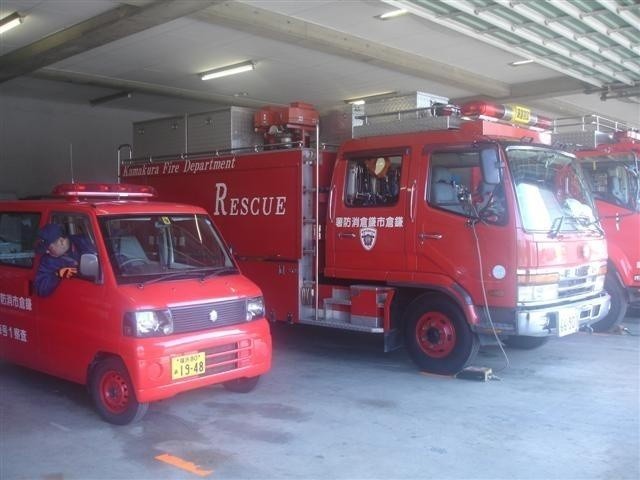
[0,183,273,426]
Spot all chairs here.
[115,235,159,266]
[433,168,504,218]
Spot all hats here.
[36,222,62,244]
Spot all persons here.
[31,221,133,299]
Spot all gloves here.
[56,267,78,279]
